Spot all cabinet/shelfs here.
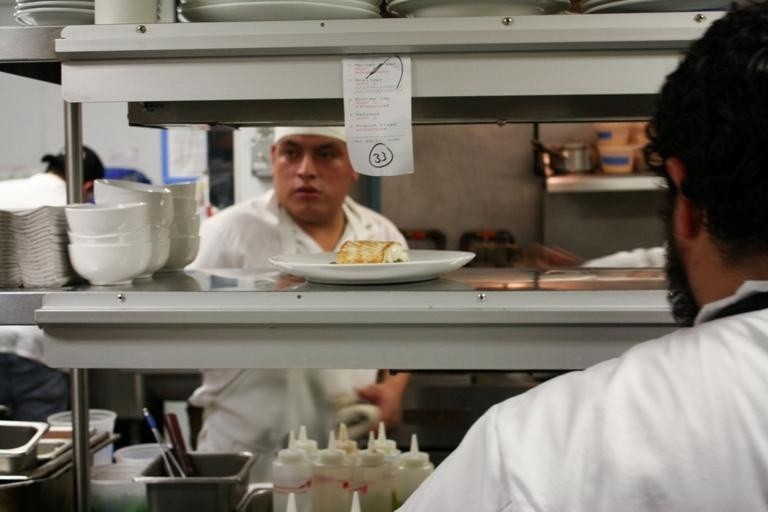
[1,9,726,510]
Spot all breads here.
[337,239,409,263]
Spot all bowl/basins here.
[65,179,201,285]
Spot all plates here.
[580,0,734,14]
[176,0,385,22]
[268,248,476,284]
[386,0,571,19]
[13,0,94,26]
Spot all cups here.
[46,408,174,512]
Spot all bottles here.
[270,421,435,512]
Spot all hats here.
[43,145,104,178]
[273,126,347,145]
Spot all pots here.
[530,136,599,177]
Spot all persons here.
[183,125,414,457]
[1,141,106,427]
[388,4,765,510]
